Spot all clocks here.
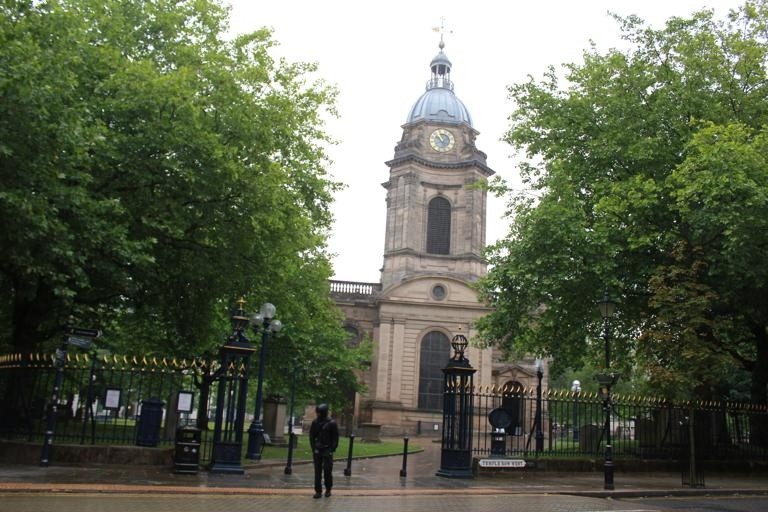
[429,128,455,153]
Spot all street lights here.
[570,378,582,442]
[244,302,283,460]
[594,284,619,491]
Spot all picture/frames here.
[175,390,194,413]
[103,387,122,410]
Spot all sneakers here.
[325,490,330,497]
[313,492,321,498]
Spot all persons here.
[308,404,340,499]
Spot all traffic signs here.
[63,335,91,350]
[52,350,65,370]
[64,324,102,337]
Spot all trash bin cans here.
[174,425,201,474]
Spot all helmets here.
[315,404,328,419]
